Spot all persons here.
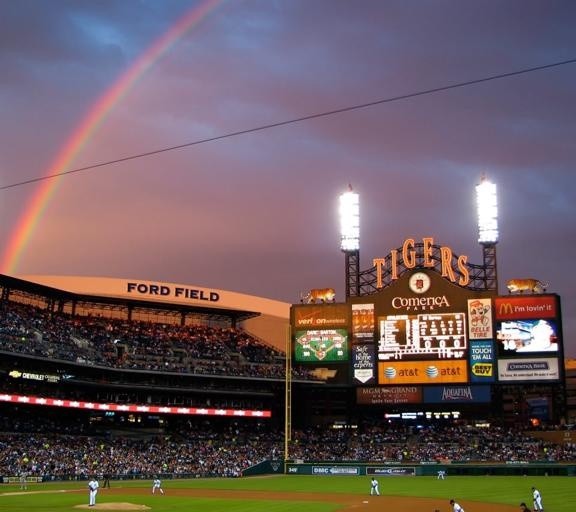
[519,502,531,512]
[101,469,112,489]
[516,319,557,351]
[314,413,575,466]
[87,477,99,506]
[18,469,29,490]
[449,499,465,512]
[532,486,544,512]
[368,477,381,496]
[1,296,313,476]
[151,476,165,496]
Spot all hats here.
[371,476,374,480]
[450,499,454,504]
[93,476,96,480]
[519,502,525,507]
